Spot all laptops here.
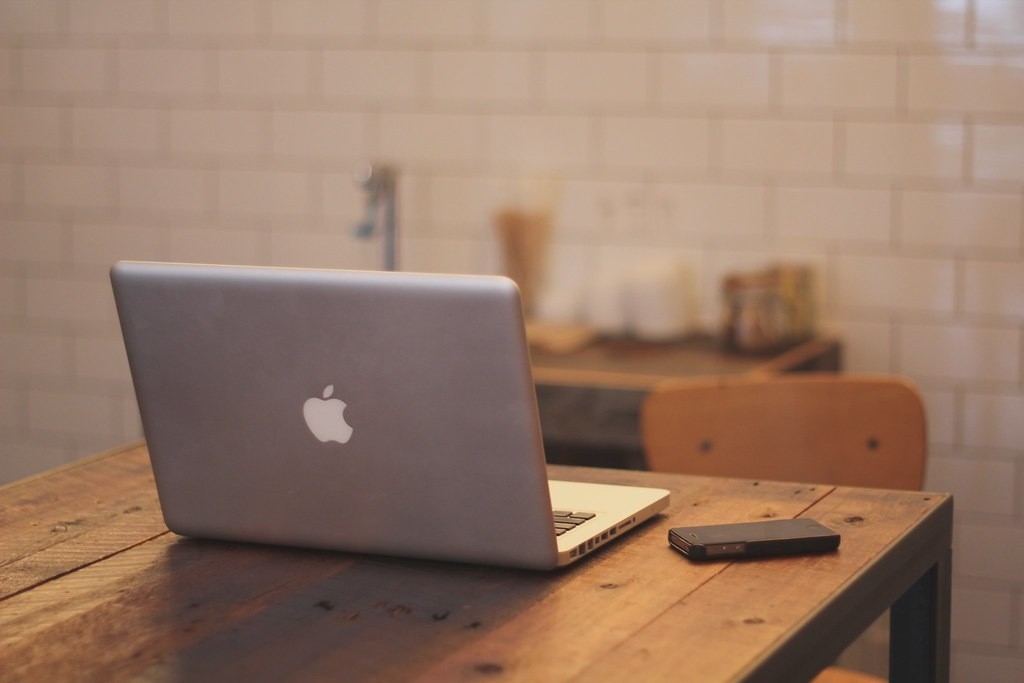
[107,260,668,571]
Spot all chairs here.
[640,375,926,494]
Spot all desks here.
[0,438,954,683]
[527,333,841,454]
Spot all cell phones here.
[666,516,841,559]
